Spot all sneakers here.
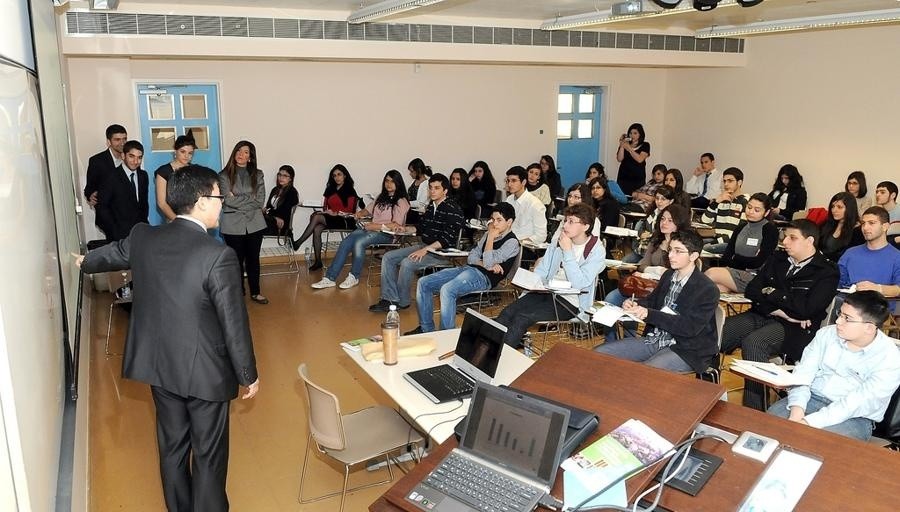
[291,236,300,253]
[403,325,422,335]
[250,294,268,305]
[310,277,337,290]
[342,272,360,291]
[368,298,400,312]
[310,261,325,271]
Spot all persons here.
[82,125,127,221]
[70,164,259,511]
[95,141,150,240]
[154,136,199,223]
[217,141,268,303]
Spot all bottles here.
[524,331,533,356]
[466,218,481,226]
[304,248,310,264]
[121,272,131,300]
[386,305,400,339]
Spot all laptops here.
[402,308,508,404]
[403,380,571,512]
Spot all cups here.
[381,322,399,365]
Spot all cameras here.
[619,134,632,142]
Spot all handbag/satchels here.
[618,273,658,299]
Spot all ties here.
[702,172,712,197]
[128,173,138,197]
[774,188,787,207]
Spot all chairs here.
[105,267,136,360]
[258,189,535,326]
[296,361,426,511]
[536,210,900,451]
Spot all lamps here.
[88,0,119,10]
[53,0,70,15]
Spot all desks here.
[338,326,536,446]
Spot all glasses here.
[786,264,800,280]
[200,194,226,201]
[834,308,873,326]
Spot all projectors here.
[610,0,665,16]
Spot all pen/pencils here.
[782,353,788,366]
[752,364,778,376]
[327,205,329,211]
[632,292,635,303]
[439,351,456,361]
